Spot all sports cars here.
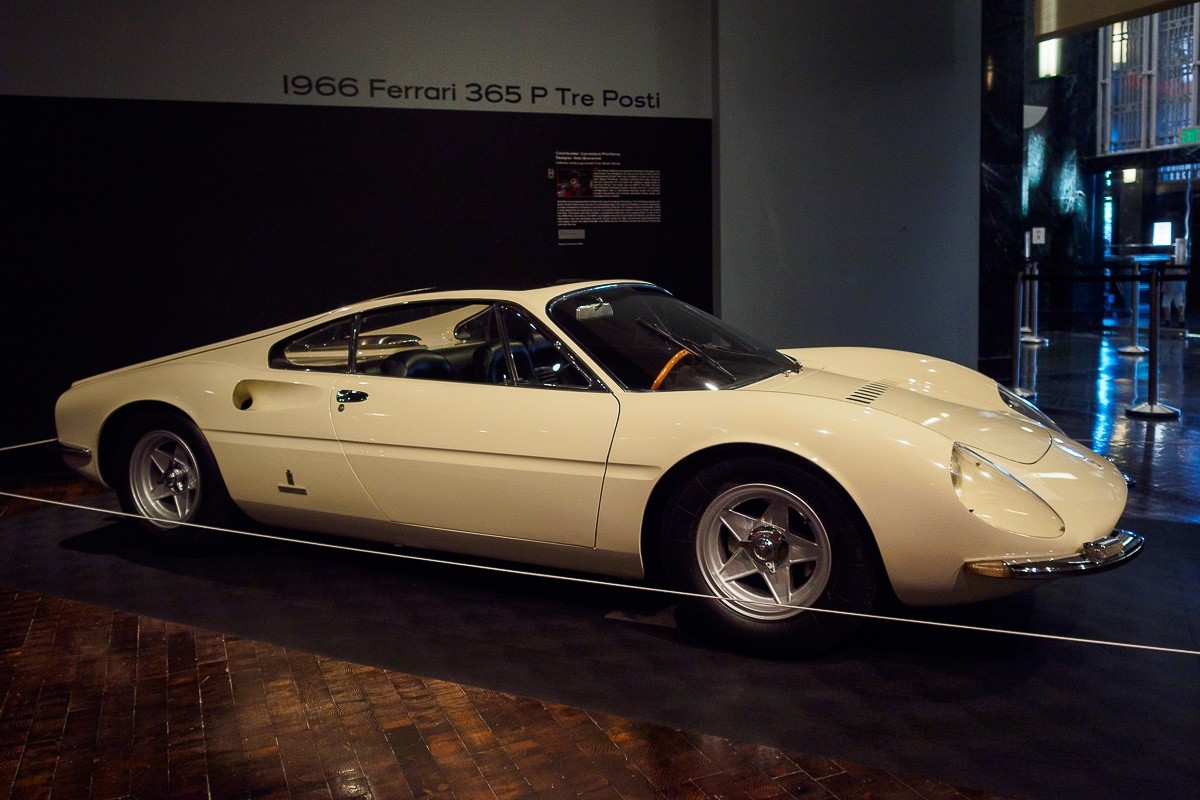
[55,279,1149,641]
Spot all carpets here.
[1,492,1200,800]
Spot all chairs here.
[478,340,540,387]
[529,341,574,387]
[382,348,455,383]
